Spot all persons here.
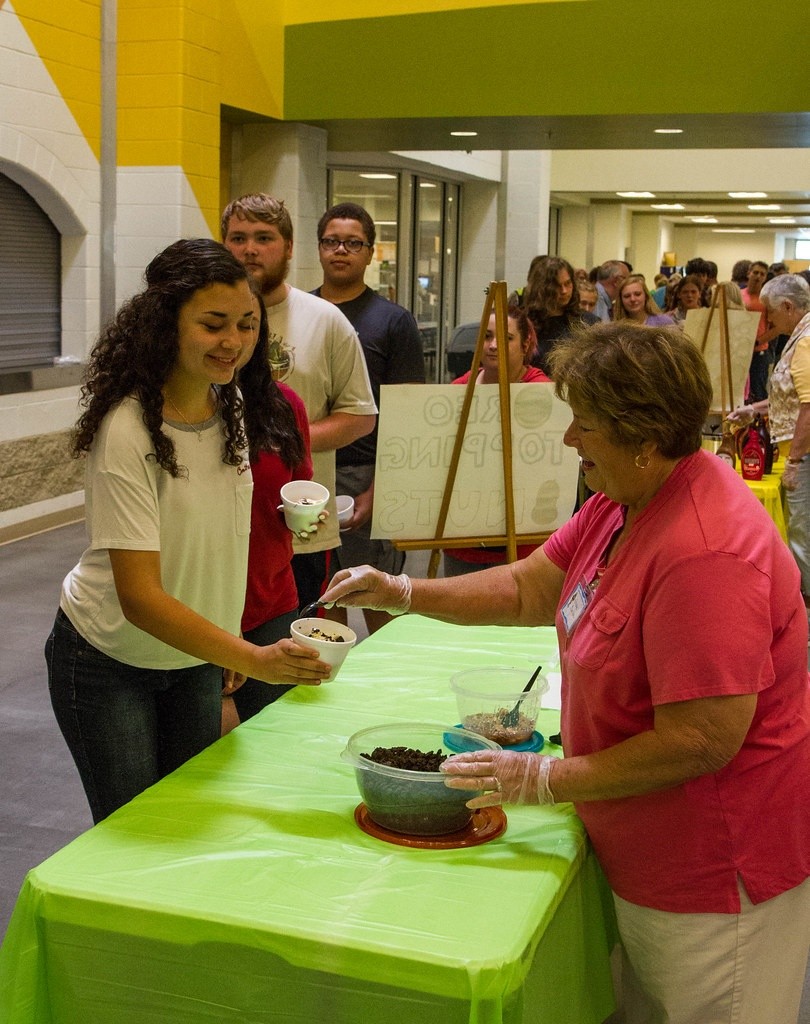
[219,193,378,619]
[443,304,552,577]
[508,255,810,646]
[45,238,332,828]
[317,322,810,1024]
[221,286,330,739]
[306,203,425,636]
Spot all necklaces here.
[162,390,208,442]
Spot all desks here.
[0,612,621,1024]
[711,454,789,552]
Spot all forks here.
[299,601,326,618]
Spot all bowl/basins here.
[449,665,550,747]
[339,721,502,836]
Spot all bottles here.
[742,422,774,475]
[740,430,765,481]
[716,435,736,471]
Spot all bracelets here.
[787,456,805,465]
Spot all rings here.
[494,777,502,793]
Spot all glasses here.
[318,236,372,254]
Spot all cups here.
[290,618,358,684]
[280,480,330,533]
[315,504,316,506]
[335,495,355,532]
